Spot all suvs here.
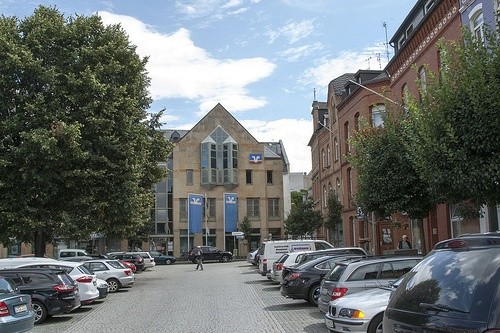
[382,231,500,333]
[317,256,424,313]
[187,246,233,264]
[1,268,82,324]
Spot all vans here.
[0,258,110,301]
[58,249,92,260]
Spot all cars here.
[15,262,99,303]
[150,251,176,265]
[99,251,155,272]
[246,240,368,283]
[280,256,375,307]
[0,277,35,333]
[83,260,134,292]
[323,272,409,333]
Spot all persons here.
[396,235,412,250]
[193,246,204,270]
